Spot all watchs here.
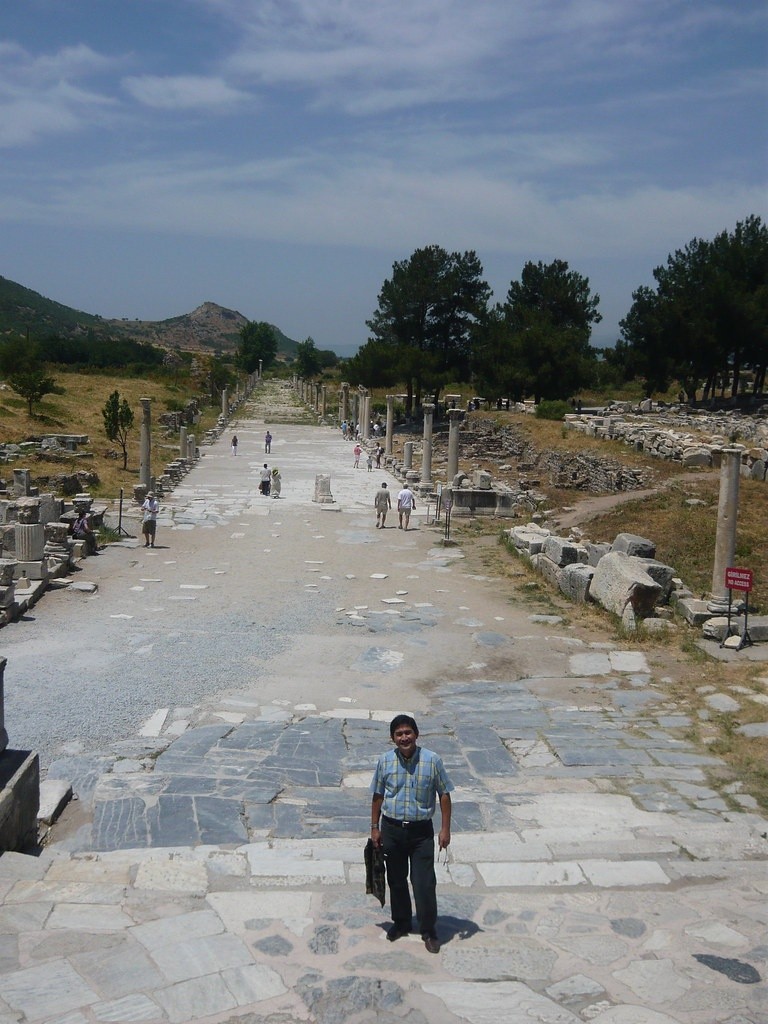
[370,823,379,828]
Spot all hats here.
[403,483,409,487]
[382,483,388,486]
[145,491,155,498]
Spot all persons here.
[141,491,159,548]
[271,470,281,498]
[71,511,99,556]
[231,436,238,455]
[340,420,380,441]
[374,482,391,528]
[369,714,456,953]
[398,484,416,531]
[354,443,363,468]
[260,463,271,496]
[366,455,373,472]
[374,442,382,469]
[265,431,272,453]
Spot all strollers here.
[259,482,272,496]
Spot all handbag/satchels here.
[72,533,77,539]
[363,834,385,908]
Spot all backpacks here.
[378,447,384,453]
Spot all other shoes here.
[376,522,379,527]
[404,527,407,531]
[387,920,412,941]
[143,542,149,547]
[398,526,402,529]
[381,526,385,528]
[87,551,98,556]
[147,543,154,548]
[421,929,439,953]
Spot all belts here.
[382,814,432,829]
